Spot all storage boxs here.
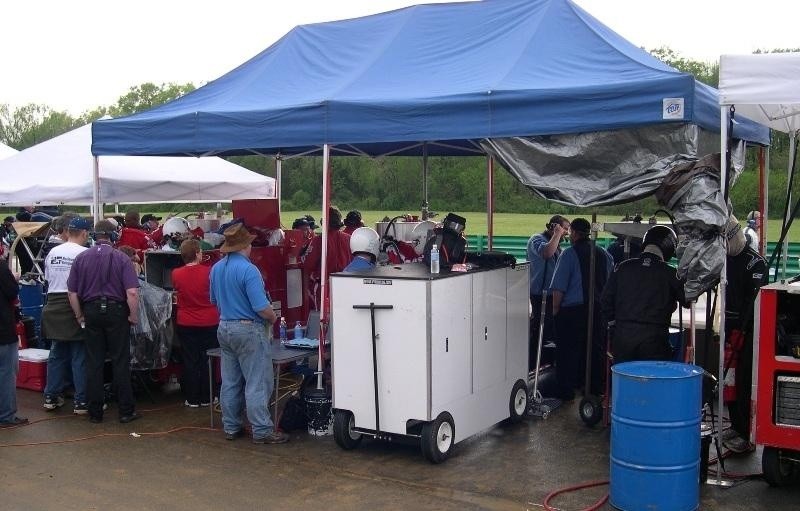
[15,348,51,392]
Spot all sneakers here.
[119,412,139,422]
[0,416,28,428]
[724,437,751,452]
[226,429,243,440]
[201,397,218,406]
[43,396,64,409]
[253,431,289,443]
[714,429,738,443]
[184,400,199,408]
[74,400,107,414]
[89,416,102,423]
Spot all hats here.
[220,222,256,252]
[141,214,162,224]
[69,217,91,230]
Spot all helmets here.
[411,222,436,256]
[641,225,678,261]
[162,216,188,236]
[350,227,380,260]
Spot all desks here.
[206,338,326,431]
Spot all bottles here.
[280,317,288,344]
[295,321,303,339]
[430,244,440,274]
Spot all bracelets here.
[75,311,85,319]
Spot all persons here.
[300,206,353,311]
[292,217,315,257]
[67,220,141,423]
[209,221,291,444]
[606,216,639,264]
[712,215,770,454]
[114,209,159,266]
[139,213,177,248]
[0,214,15,230]
[301,214,319,236]
[42,216,97,417]
[379,234,421,264]
[741,209,763,252]
[0,230,17,258]
[342,227,380,272]
[527,214,571,370]
[0,230,29,428]
[16,206,38,279]
[547,217,613,402]
[170,238,222,408]
[40,212,110,414]
[600,224,693,366]
[343,210,365,235]
